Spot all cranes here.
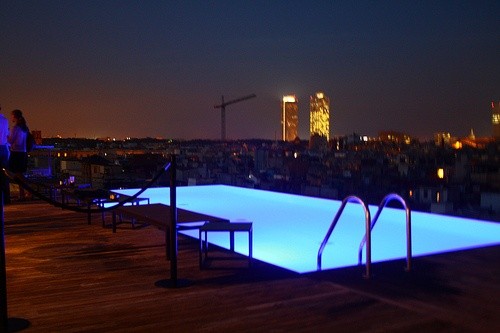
[215,93,256,141]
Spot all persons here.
[0,105,12,205]
[7,110,29,202]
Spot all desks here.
[61,189,252,270]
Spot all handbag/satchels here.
[26,131,34,151]
